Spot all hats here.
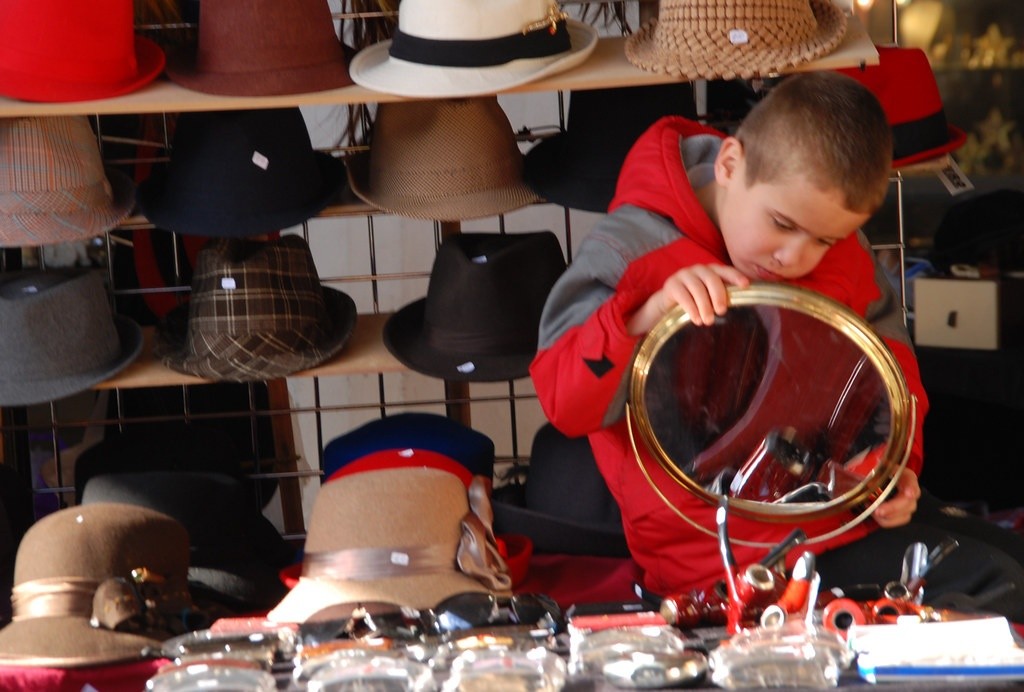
[0,0,979,671]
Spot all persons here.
[528,67,1024,626]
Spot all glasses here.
[428,589,565,633]
[298,596,427,651]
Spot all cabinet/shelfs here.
[0,12,880,396]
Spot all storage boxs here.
[912,278,1002,349]
